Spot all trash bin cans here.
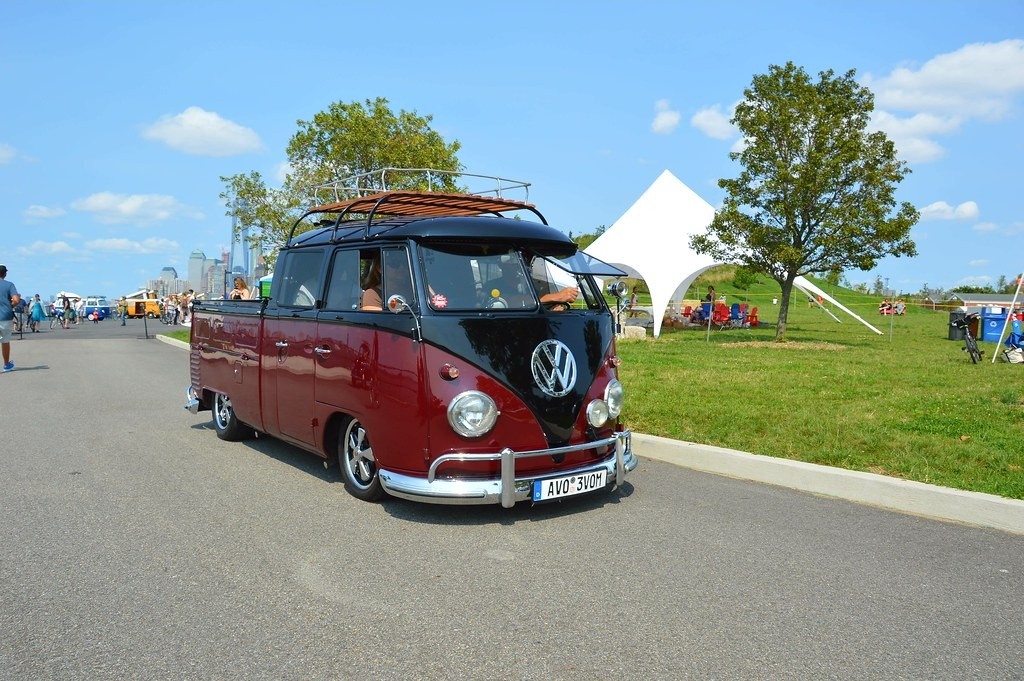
[948,313,966,340]
[965,314,980,339]
[981,306,1006,343]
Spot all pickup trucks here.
[182,166,642,511]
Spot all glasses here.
[384,259,408,269]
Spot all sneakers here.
[3,360,14,371]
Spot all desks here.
[610,306,685,316]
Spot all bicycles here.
[51,308,69,329]
[947,312,985,364]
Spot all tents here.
[472,256,604,309]
[249,271,316,307]
[559,170,885,339]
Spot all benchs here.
[880,306,910,315]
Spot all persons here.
[51,293,85,329]
[94,308,98,324]
[628,286,639,319]
[286,250,436,310]
[120,296,128,326]
[0,265,20,371]
[719,293,727,301]
[12,294,48,334]
[773,296,778,305]
[481,252,579,311]
[817,295,823,309]
[879,298,892,316]
[894,299,906,316]
[807,293,815,309]
[690,285,716,327]
[159,289,196,325]
[219,277,250,299]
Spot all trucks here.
[77,296,110,321]
[118,290,166,319]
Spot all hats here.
[498,248,537,269]
[59,291,65,296]
[122,295,126,299]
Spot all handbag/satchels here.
[1000,342,1024,364]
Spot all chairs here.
[675,303,759,332]
